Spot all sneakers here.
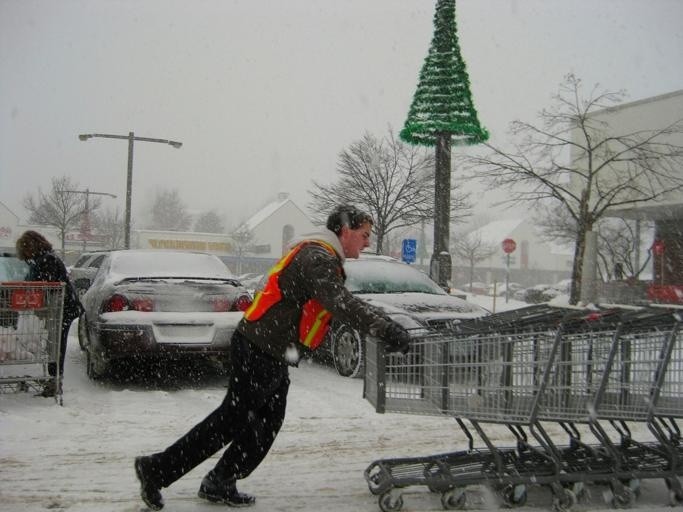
[198,471,256,508]
[135,456,163,511]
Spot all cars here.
[463,277,680,306]
[66,250,105,294]
[77,250,253,384]
[255,247,509,386]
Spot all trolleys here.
[0,277,68,406]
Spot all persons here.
[133,202,412,512]
[14,230,86,397]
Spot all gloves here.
[381,327,410,355]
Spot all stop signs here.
[503,238,516,253]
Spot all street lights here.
[79,131,182,249]
[59,189,117,252]
